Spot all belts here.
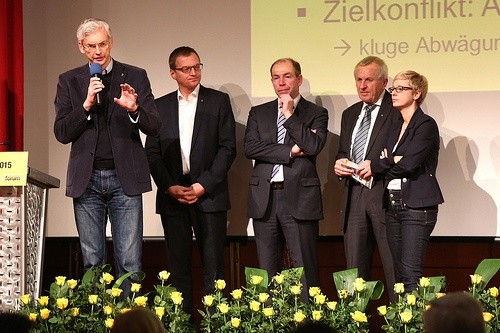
[270,182,284,189]
[352,181,364,187]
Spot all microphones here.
[89,63,103,106]
[0,141,9,145]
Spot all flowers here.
[17,258,500,333]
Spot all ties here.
[352,104,376,164]
[271,111,286,178]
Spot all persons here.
[144,46,236,333]
[244,58,329,333]
[53,18,162,305]
[111,307,165,333]
[424,295,485,333]
[334,56,403,333]
[388,70,445,333]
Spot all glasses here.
[82,42,110,52]
[388,86,413,94]
[175,63,203,73]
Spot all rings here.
[341,162,347,167]
